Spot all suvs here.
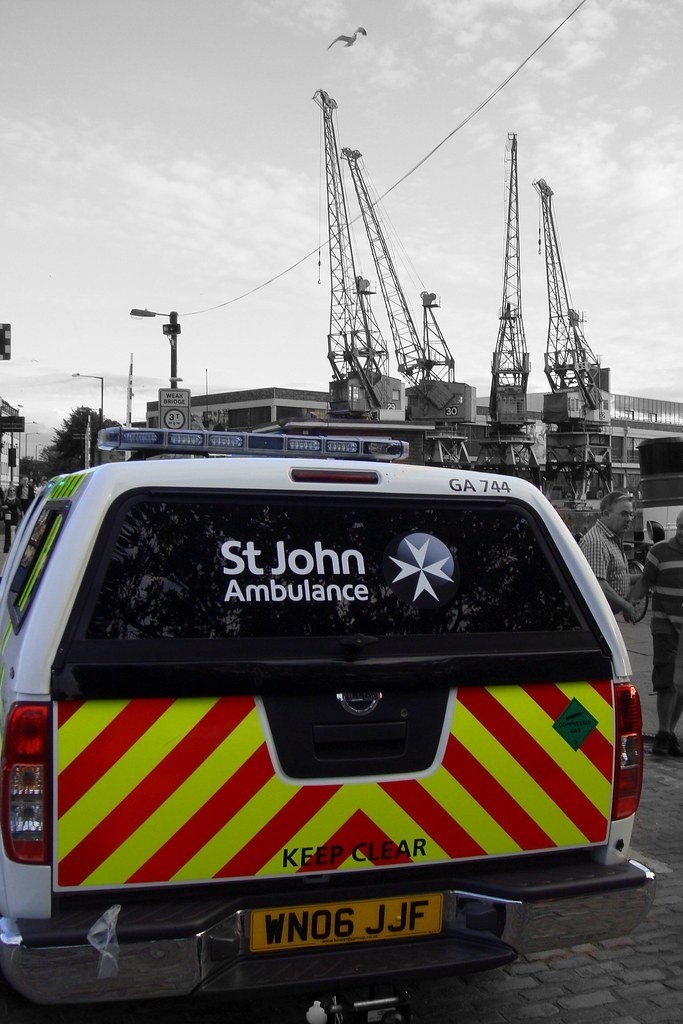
[0,420,661,1024]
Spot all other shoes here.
[651,730,670,756]
[670,732,682,757]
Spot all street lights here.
[71,370,105,421]
[126,305,180,389]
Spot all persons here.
[623,509,683,758]
[578,491,639,627]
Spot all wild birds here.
[326,26,366,50]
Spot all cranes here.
[487,130,537,425]
[337,147,480,423]
[314,90,406,420]
[531,175,616,429]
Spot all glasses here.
[622,510,637,518]
[617,492,635,502]
